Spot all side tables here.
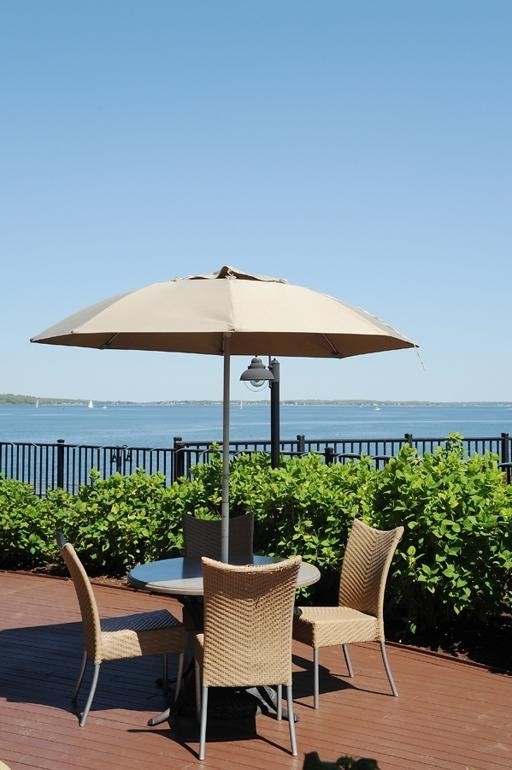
[127,556,322,726]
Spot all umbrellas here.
[30,266,420,564]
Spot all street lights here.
[240,354,280,470]
[112,445,131,476]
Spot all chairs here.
[180,512,255,556]
[291,517,405,709]
[193,556,303,760]
[59,543,192,728]
